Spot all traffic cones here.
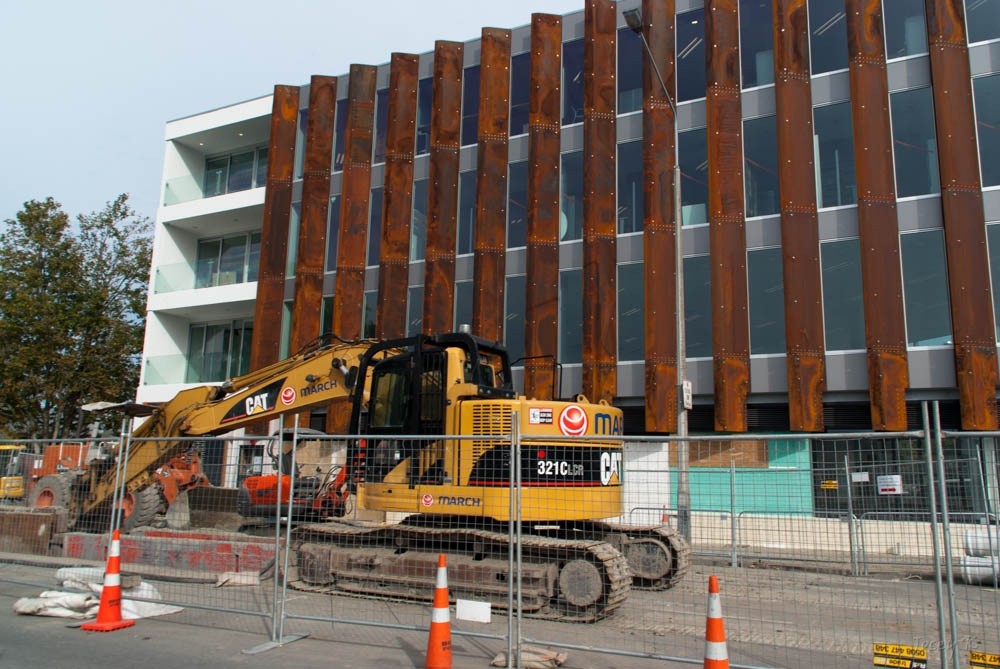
[425,554,453,669]
[81,529,135,633]
[702,576,731,669]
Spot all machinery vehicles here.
[0,441,357,529]
[61,324,690,623]
